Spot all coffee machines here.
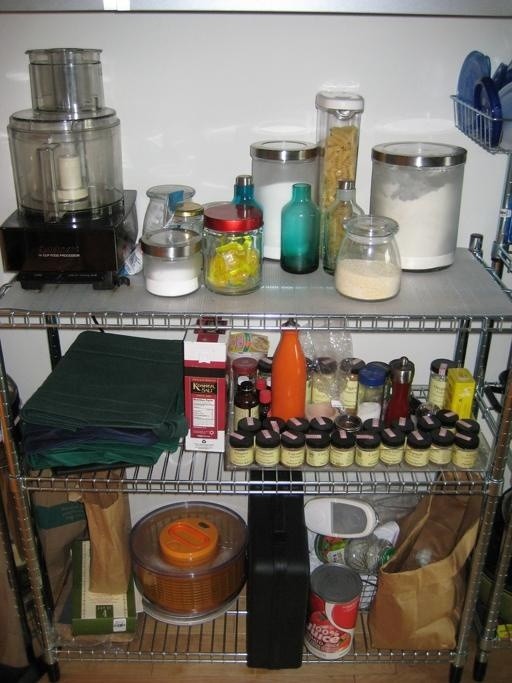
[0,45,134,288]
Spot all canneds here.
[259,358,276,384]
[304,356,314,403]
[426,359,454,408]
[227,412,480,473]
[303,561,362,659]
[202,204,262,295]
[315,357,334,404]
[339,359,364,407]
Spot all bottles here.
[313,533,397,575]
[372,139,469,271]
[313,88,366,247]
[326,177,365,274]
[247,141,326,264]
[227,354,480,470]
[270,319,306,418]
[278,180,321,273]
[335,215,401,301]
[136,172,266,293]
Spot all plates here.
[138,596,239,626]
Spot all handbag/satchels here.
[29,469,139,644]
[369,470,483,650]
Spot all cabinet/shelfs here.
[447,89,512,681]
[1,231,512,681]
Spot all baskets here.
[452,94,512,155]
